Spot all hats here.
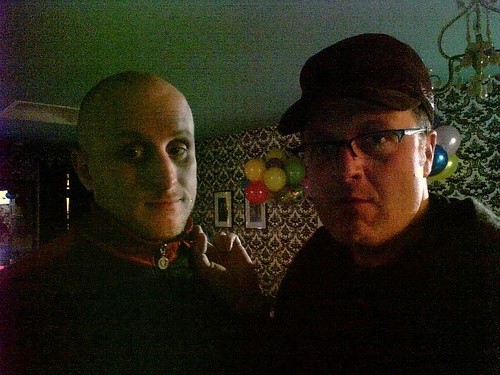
[277,33,435,135]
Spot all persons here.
[0,72,265,375]
[246,32,500,375]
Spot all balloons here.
[427,126,462,181]
[244,148,306,205]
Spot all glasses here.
[290,128,432,170]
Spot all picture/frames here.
[243,195,268,229]
[214,190,233,228]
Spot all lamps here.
[428,0,500,96]
